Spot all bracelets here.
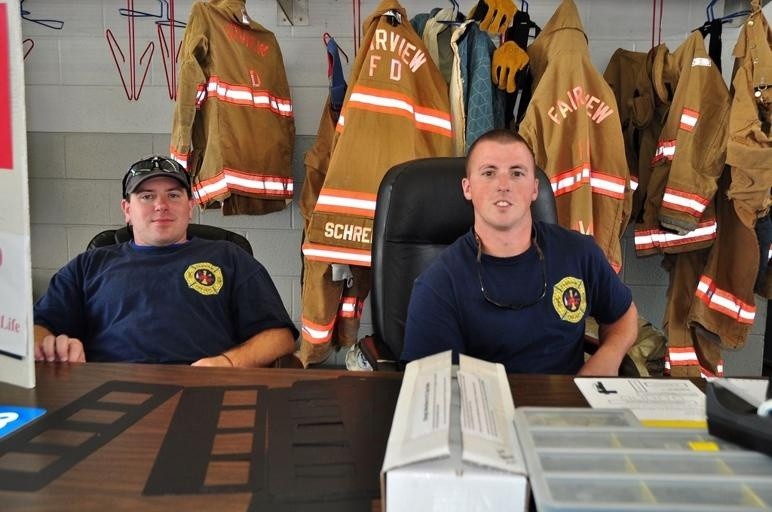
[220,351,235,368]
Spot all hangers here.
[686,1,757,37]
[361,1,547,44]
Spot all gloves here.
[468,0,517,34]
[491,42,531,92]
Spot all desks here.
[0,361,771,510]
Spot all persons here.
[26,154,298,368]
[399,127,641,376]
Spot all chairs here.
[82,226,305,370]
[345,155,643,379]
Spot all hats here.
[124,156,190,196]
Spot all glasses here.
[128,159,180,174]
